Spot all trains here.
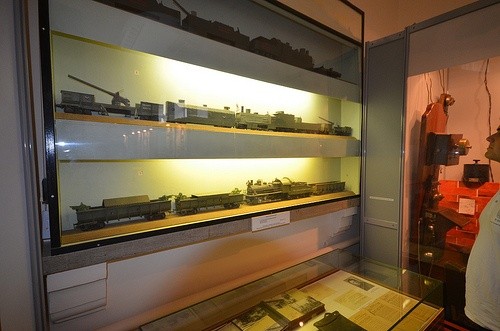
[55,1,353,232]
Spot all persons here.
[348,278,375,291]
[464,125,500,331]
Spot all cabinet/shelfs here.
[0,0,366,331]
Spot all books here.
[207,303,290,331]
[260,287,326,330]
